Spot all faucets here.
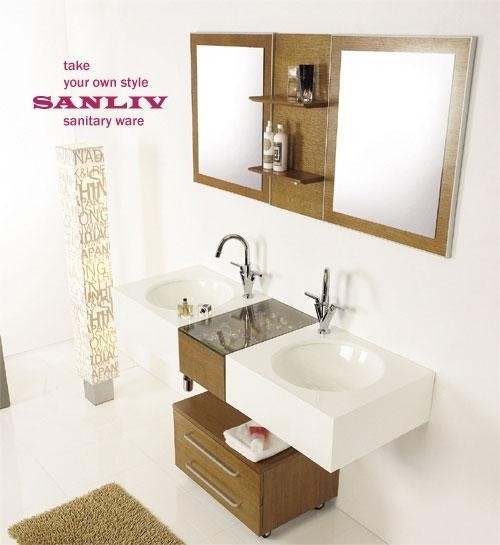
[213,234,262,301]
[304,268,344,334]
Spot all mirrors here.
[189,32,271,207]
[325,34,476,260]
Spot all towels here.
[223,419,291,463]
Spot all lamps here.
[56,139,118,406]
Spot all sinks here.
[145,278,234,311]
[225,323,438,476]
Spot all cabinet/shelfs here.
[247,94,327,187]
[170,393,341,541]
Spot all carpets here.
[7,482,188,545]
